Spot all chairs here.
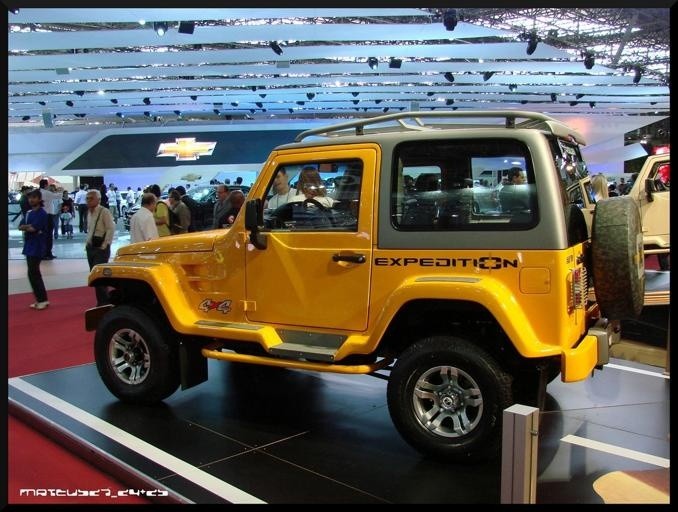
[399,173,472,227]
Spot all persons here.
[15,176,249,242]
[84,189,115,309]
[265,167,299,209]
[18,188,51,312]
[508,166,525,187]
[287,166,341,210]
[590,173,638,205]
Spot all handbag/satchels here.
[157,201,179,233]
[91,236,103,247]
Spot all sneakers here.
[29,301,49,309]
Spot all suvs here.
[80,108,650,462]
[122,185,250,231]
[602,149,670,250]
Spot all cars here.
[471,182,500,214]
